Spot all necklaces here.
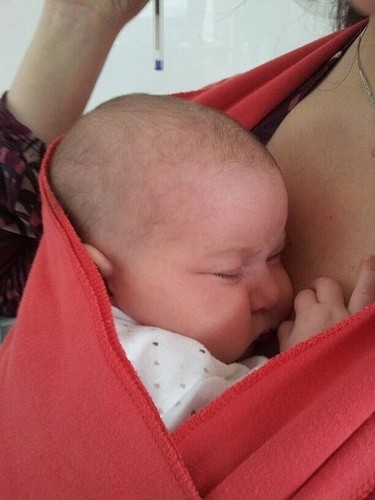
[354,25,375,105]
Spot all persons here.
[20,93,375,499]
[0,0,375,499]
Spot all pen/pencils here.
[153,1,163,70]
